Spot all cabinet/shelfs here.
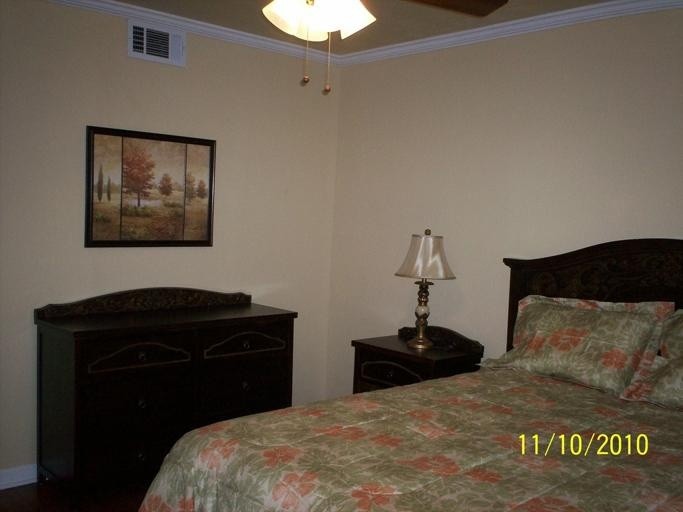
[37,302,300,503]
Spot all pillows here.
[494,292,683,409]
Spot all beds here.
[140,236,683,512]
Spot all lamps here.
[393,227,456,352]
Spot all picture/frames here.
[85,126,216,247]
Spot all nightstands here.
[351,326,487,395]
